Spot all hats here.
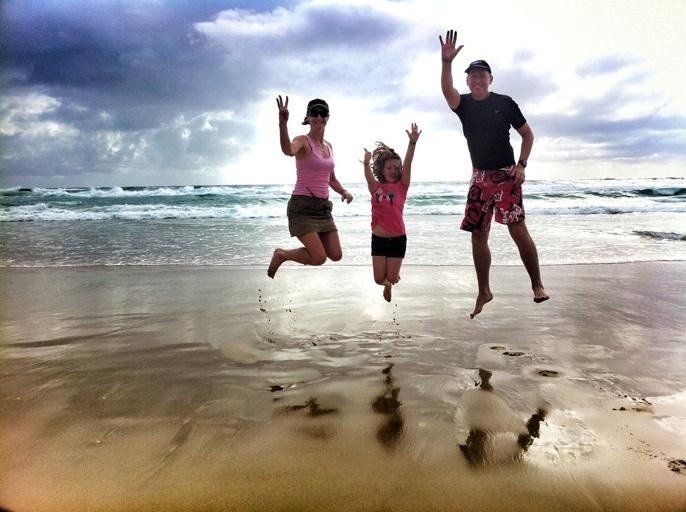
[307,99,329,112]
[464,60,491,74]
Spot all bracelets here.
[340,189,347,194]
[363,161,370,165]
[409,141,417,145]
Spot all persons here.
[362,122,422,303]
[267,94,354,280]
[439,28,550,318]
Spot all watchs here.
[517,159,528,167]
